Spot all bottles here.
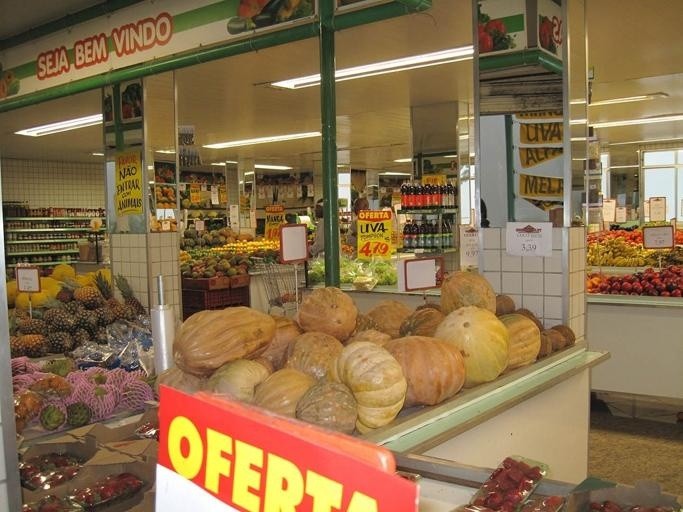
[54,255,80,261]
[30,207,106,217]
[4,221,32,229]
[403,218,453,248]
[2,199,29,217]
[6,242,78,253]
[12,256,53,263]
[7,233,54,241]
[33,221,106,228]
[401,181,456,209]
[54,232,105,239]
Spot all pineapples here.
[10,270,149,363]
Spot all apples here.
[587,228,683,245]
[587,265,683,297]
[210,237,280,254]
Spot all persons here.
[348,198,370,260]
[382,199,398,230]
[307,198,324,258]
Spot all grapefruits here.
[6,264,112,311]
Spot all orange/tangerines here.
[187,248,218,259]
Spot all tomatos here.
[19,452,141,512]
[450,457,683,512]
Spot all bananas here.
[585,236,672,269]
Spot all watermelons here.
[180,228,227,251]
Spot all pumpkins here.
[222,227,253,242]
[155,271,541,436]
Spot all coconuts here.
[497,295,576,358]
